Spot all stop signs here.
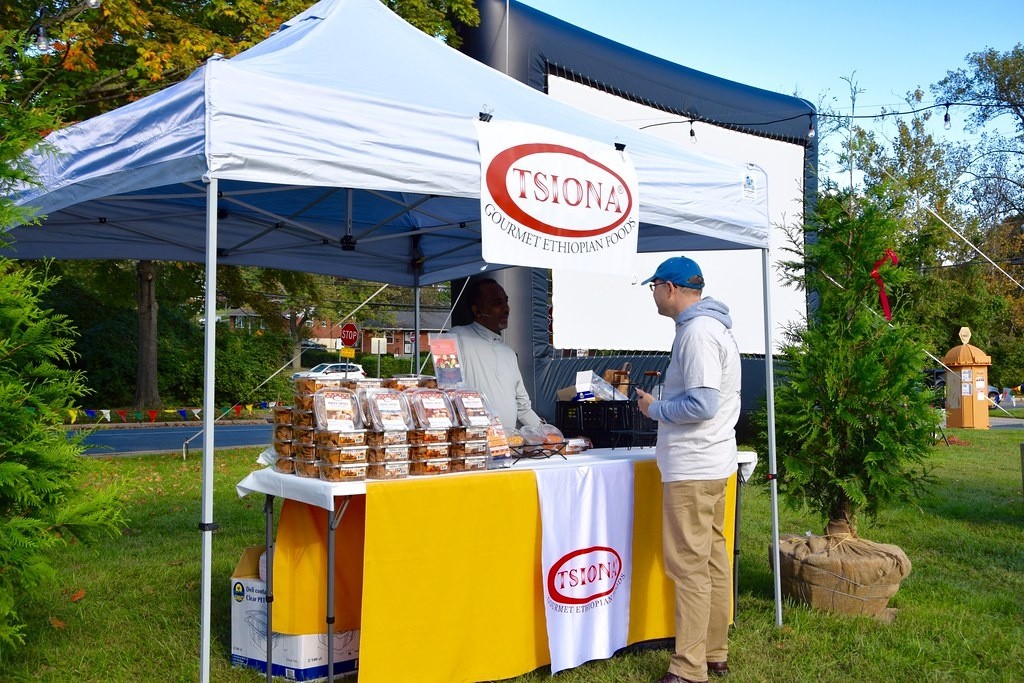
[341,323,359,347]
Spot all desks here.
[235,446,756,683]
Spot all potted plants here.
[747,67,945,623]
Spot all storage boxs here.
[555,369,658,448]
[270,374,585,483]
[229,547,361,683]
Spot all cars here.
[289,362,366,388]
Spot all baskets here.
[555,401,658,448]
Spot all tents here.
[0,0,783,683]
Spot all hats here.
[641,256,705,289]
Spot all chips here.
[273,379,490,481]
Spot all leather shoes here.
[706,662,728,676]
[654,672,708,683]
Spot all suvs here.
[919,369,1000,409]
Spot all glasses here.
[649,282,677,291]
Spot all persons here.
[636,256,741,683]
[448,279,545,429]
